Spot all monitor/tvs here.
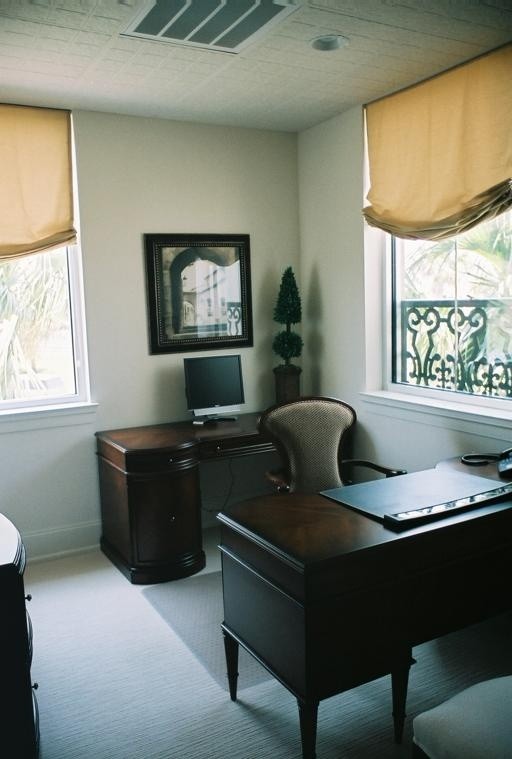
[184,353,247,425]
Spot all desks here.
[215,453,512,758]
[94,413,357,585]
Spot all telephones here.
[497,448,512,475]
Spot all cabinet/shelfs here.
[0,512,42,759]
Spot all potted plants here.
[273,266,303,406]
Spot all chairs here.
[413,676,512,759]
[264,455,407,495]
[258,396,356,494]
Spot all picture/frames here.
[144,231,253,356]
